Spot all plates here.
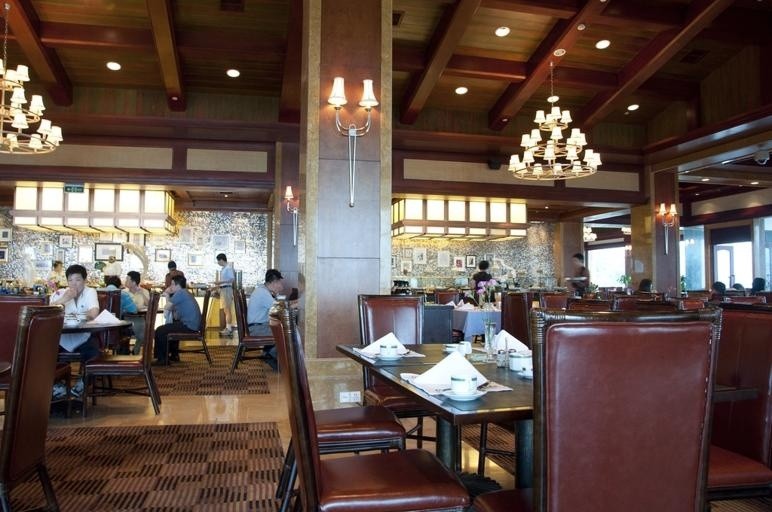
[374,353,404,360]
[518,371,533,379]
[442,343,459,349]
[442,390,486,401]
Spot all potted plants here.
[618,272,635,296]
[583,282,598,300]
[679,275,689,299]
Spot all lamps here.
[655,201,679,251]
[13,177,179,235]
[396,197,526,244]
[329,77,378,207]
[285,185,299,215]
[0,3,63,153]
[510,59,601,183]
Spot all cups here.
[76,313,87,319]
[64,315,74,320]
[495,292,501,310]
[66,318,80,325]
[450,375,478,395]
[379,345,397,357]
[459,341,472,354]
[509,353,533,371]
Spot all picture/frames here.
[392,255,397,268]
[413,248,426,264]
[401,261,411,272]
[58,234,73,248]
[454,257,466,272]
[127,233,146,253]
[95,242,123,261]
[438,251,450,266]
[0,228,12,241]
[0,246,8,263]
[466,255,476,268]
[484,253,494,267]
[155,249,172,262]
[403,248,412,258]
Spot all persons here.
[471,261,495,307]
[748,277,766,297]
[154,277,202,365]
[565,253,590,294]
[47,261,64,287]
[50,268,99,398]
[732,283,744,293]
[165,262,186,291]
[102,248,122,277]
[246,268,284,371]
[120,270,151,315]
[707,281,730,302]
[216,254,235,337]
[638,279,654,295]
[97,277,137,319]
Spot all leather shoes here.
[264,353,279,371]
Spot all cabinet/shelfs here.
[141,284,167,313]
[423,305,454,344]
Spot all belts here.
[246,322,264,328]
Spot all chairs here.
[622,302,677,312]
[727,296,766,305]
[166,287,212,364]
[476,310,724,512]
[269,307,470,511]
[57,290,112,361]
[0,296,45,390]
[540,292,554,307]
[270,304,401,512]
[110,289,130,354]
[682,287,714,300]
[724,290,745,297]
[680,300,704,310]
[82,292,161,415]
[756,292,772,302]
[0,303,65,512]
[570,302,610,313]
[502,291,532,347]
[359,293,438,454]
[544,295,568,310]
[434,291,460,306]
[607,289,664,309]
[705,310,772,512]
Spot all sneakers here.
[219,328,233,336]
[151,358,165,367]
[70,380,84,398]
[52,385,68,399]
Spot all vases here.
[481,303,500,353]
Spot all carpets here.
[460,421,516,475]
[8,422,296,512]
[89,345,270,395]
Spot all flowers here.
[464,280,498,309]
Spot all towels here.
[491,329,530,354]
[413,352,489,395]
[362,332,408,358]
[91,308,122,325]
